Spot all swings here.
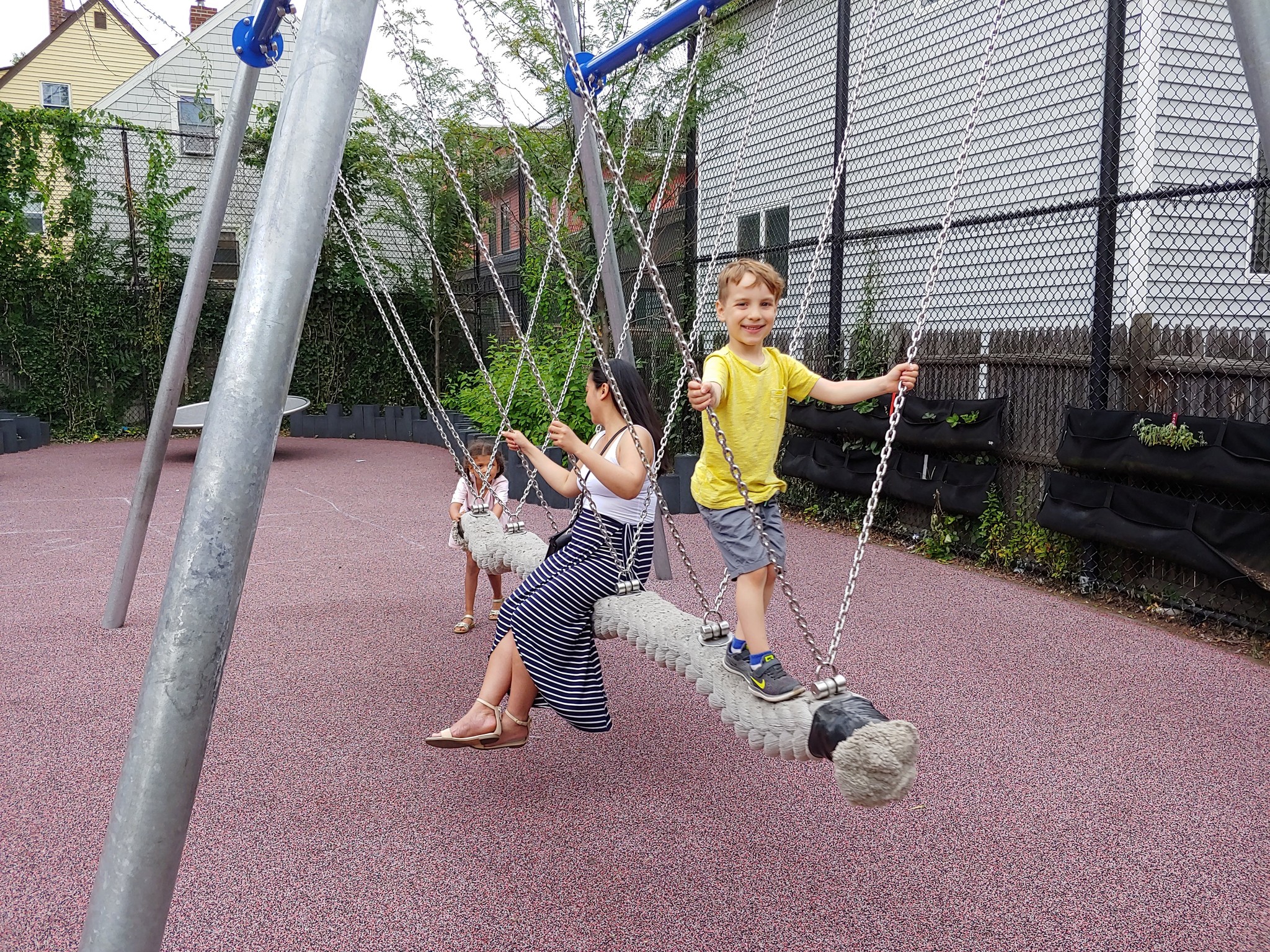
[257,0,1007,812]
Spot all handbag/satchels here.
[543,519,574,560]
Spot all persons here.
[425,361,672,751]
[448,442,508,632]
[687,258,920,703]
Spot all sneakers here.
[747,653,807,702]
[722,639,751,684]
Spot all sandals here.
[425,697,502,749]
[469,708,534,750]
[489,597,506,620]
[454,614,476,634]
